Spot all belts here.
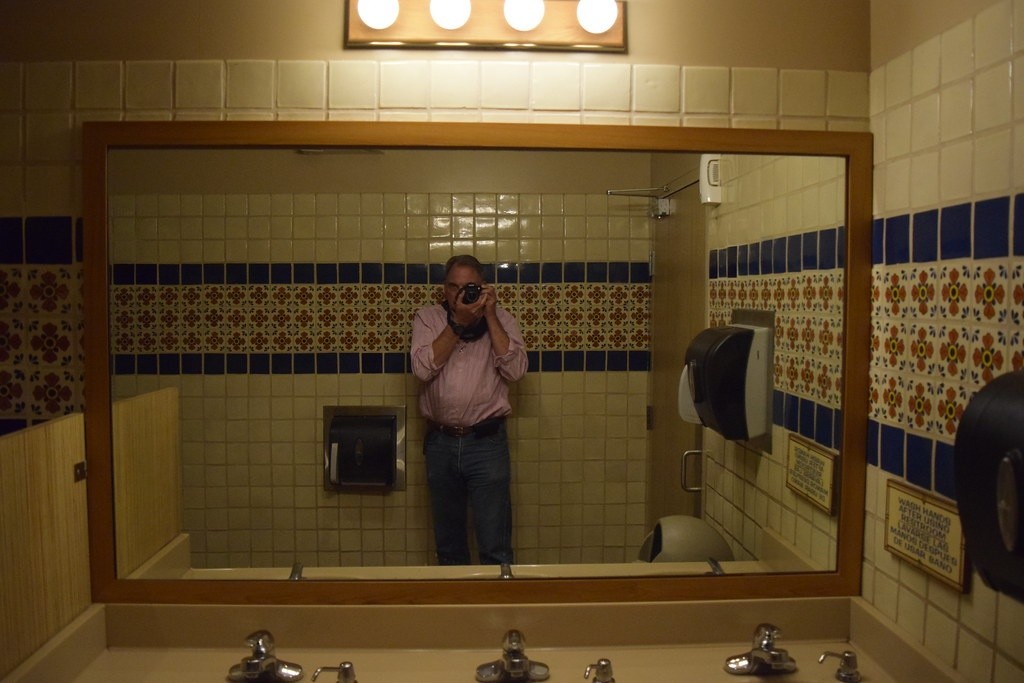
[427,416,503,436]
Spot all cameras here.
[462,282,482,304]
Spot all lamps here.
[344,0,628,53]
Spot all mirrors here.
[81,120,875,602]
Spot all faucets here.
[289,562,306,580]
[499,563,514,579]
[704,557,725,575]
[226,628,303,682]
[475,628,550,681]
[723,621,797,674]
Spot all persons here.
[411,254,528,566]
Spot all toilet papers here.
[676,363,703,424]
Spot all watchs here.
[449,320,463,335]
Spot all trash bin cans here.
[637,514,735,563]
